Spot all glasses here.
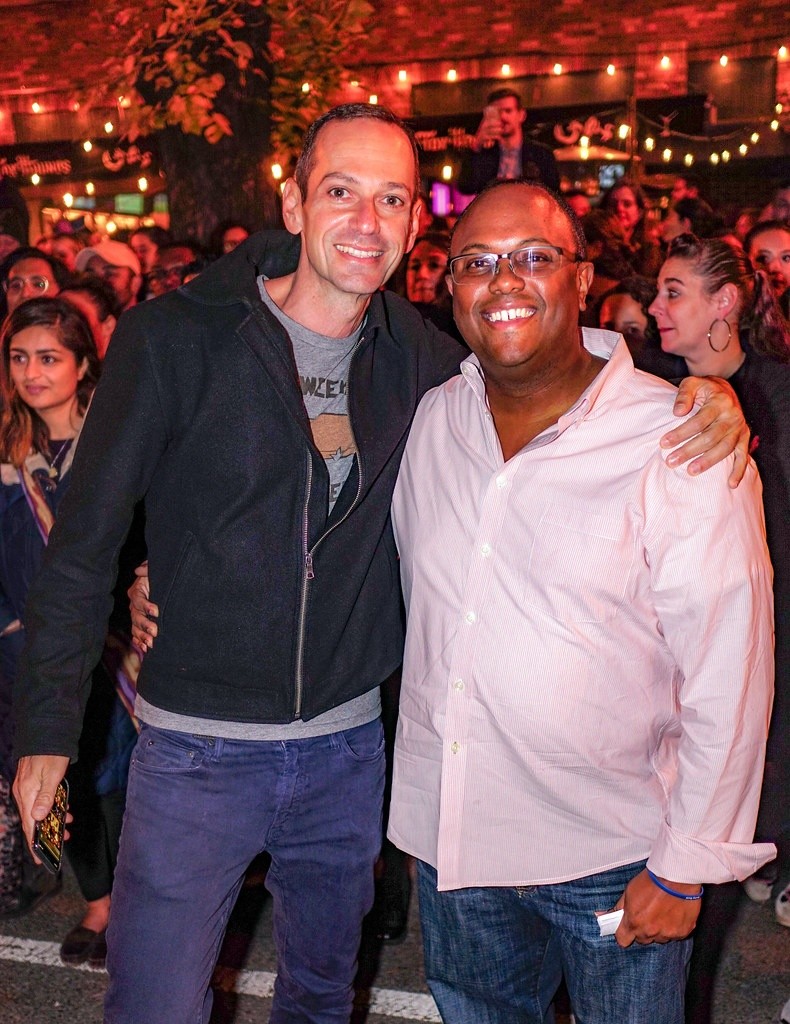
[146,261,201,293]
[446,245,583,286]
[1,275,55,298]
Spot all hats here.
[73,240,141,276]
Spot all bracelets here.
[647,869,704,901]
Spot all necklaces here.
[38,431,75,493]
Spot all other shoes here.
[744,877,774,903]
[775,882,790,928]
[368,870,413,944]
[59,923,109,966]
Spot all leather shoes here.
[0,863,64,916]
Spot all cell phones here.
[31,775,69,876]
[483,108,501,136]
[600,199,618,220]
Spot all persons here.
[10,107,750,1024]
[127,179,777,1024]
[0,83,790,1024]
